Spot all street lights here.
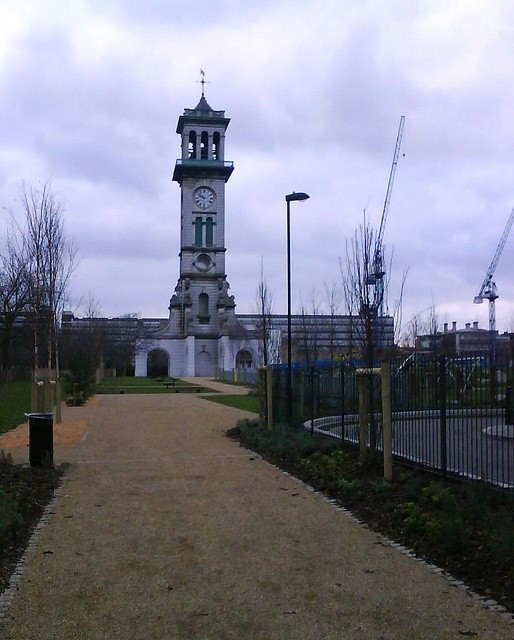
[285,191,310,421]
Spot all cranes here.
[475,207,514,365]
[360,116,406,315]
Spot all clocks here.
[194,189,214,208]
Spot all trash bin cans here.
[29,411,53,469]
